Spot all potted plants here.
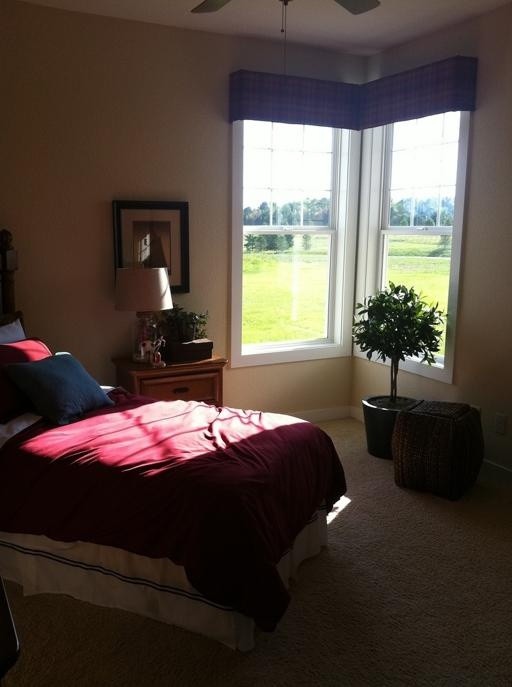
[161,302,213,359]
[352,280,449,461]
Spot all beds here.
[0,230,329,652]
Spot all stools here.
[393,399,485,501]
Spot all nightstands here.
[111,349,229,410]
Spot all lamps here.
[115,267,174,368]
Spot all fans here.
[190,0,382,17]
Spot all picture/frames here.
[112,199,193,295]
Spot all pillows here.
[0,317,113,426]
